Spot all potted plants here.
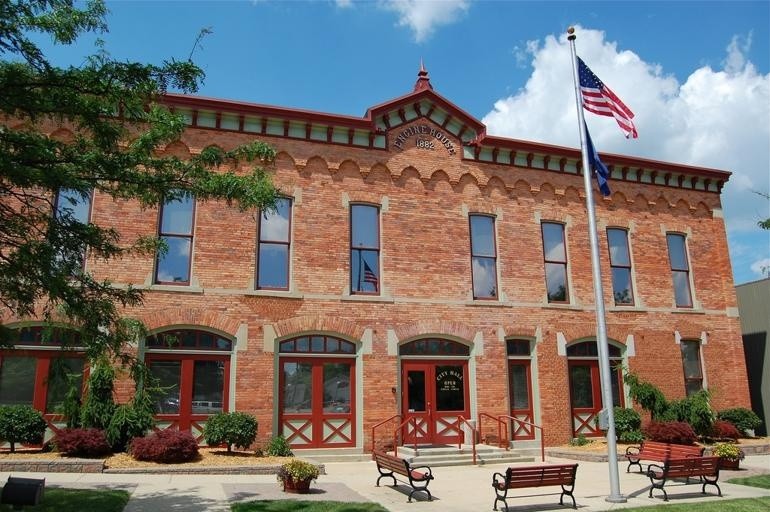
[277,459,322,496]
[711,438,747,471]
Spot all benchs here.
[369,447,581,511]
[623,440,726,505]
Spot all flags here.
[363,261,378,293]
[577,53,638,139]
[585,121,612,198]
